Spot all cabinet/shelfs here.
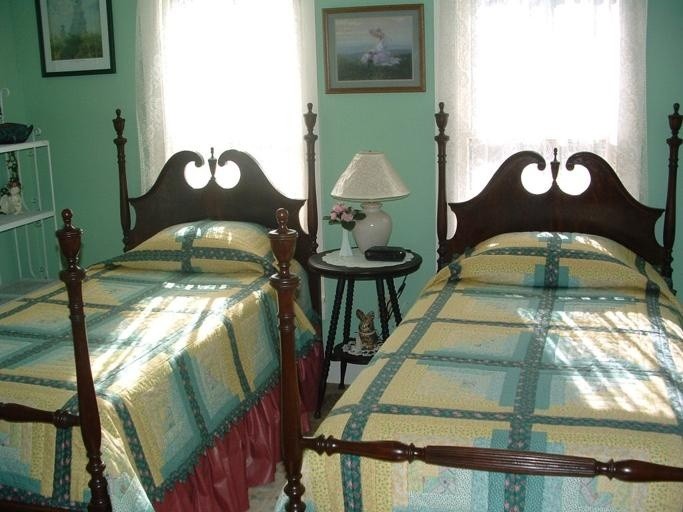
[0,87,62,308]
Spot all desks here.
[308,245,424,419]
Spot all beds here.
[0,99,321,511]
[271,101,682,512]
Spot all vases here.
[338,230,357,259]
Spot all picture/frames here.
[320,3,427,96]
[34,1,117,78]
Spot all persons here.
[361,26,398,67]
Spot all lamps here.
[328,148,413,258]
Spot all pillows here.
[420,224,683,308]
[103,217,278,276]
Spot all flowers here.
[323,202,362,230]
